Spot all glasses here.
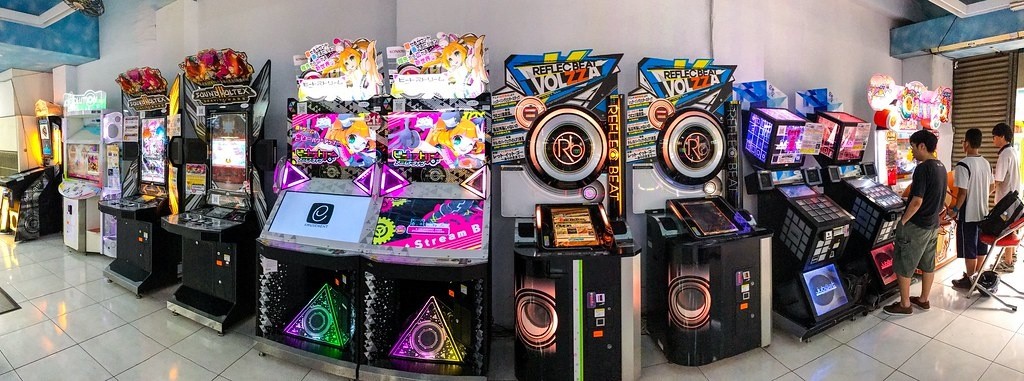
[961,140,966,144]
[993,136,998,140]
[910,143,917,150]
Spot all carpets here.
[0,287,22,315]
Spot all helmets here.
[977,270,1000,297]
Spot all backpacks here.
[979,190,1024,235]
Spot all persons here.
[883,129,948,315]
[991,123,1021,271]
[943,129,994,289]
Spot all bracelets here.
[952,206,960,212]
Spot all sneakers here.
[883,301,913,316]
[990,260,1015,272]
[996,253,1017,262]
[910,296,931,310]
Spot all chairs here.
[967,217,1024,310]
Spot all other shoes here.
[952,271,971,288]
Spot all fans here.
[63,0,105,17]
